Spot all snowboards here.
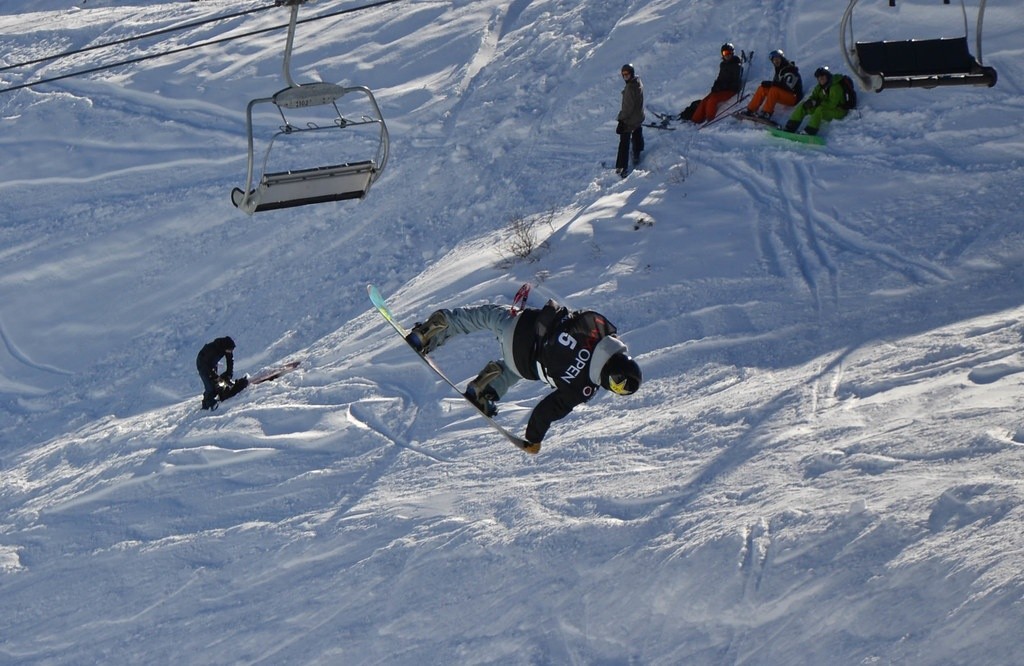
[727,110,779,126]
[248,360,301,385]
[366,283,536,453]
[767,127,827,145]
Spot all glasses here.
[722,50,732,55]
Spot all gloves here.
[616,122,625,134]
[802,100,814,110]
[761,80,772,86]
[524,441,541,454]
[220,373,232,379]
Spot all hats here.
[225,336,236,350]
[623,67,630,75]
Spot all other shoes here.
[741,110,752,116]
[797,129,816,135]
[777,125,795,133]
[467,380,495,417]
[755,111,771,120]
[406,334,421,349]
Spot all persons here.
[406,302,641,453]
[778,66,848,137]
[195,336,236,410]
[615,64,645,174]
[691,43,743,124]
[741,48,804,118]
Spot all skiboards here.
[641,105,681,132]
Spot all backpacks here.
[825,75,856,109]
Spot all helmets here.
[621,64,635,78]
[814,67,832,83]
[602,352,642,396]
[721,43,734,56]
[768,50,785,64]
